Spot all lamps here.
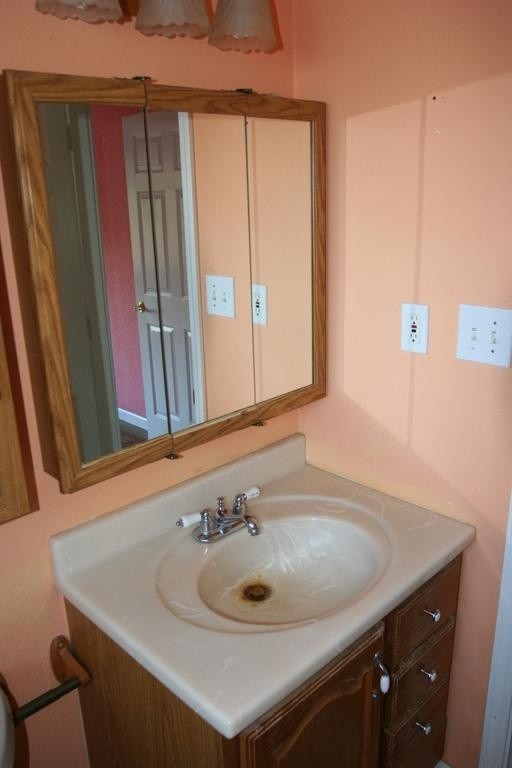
[35,0,277,55]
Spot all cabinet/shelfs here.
[63,547,463,767]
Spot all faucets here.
[217,499,259,537]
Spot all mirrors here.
[2,70,327,494]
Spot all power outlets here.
[252,284,267,325]
[401,303,427,353]
[205,275,235,320]
[455,304,512,368]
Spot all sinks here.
[199,515,378,627]
